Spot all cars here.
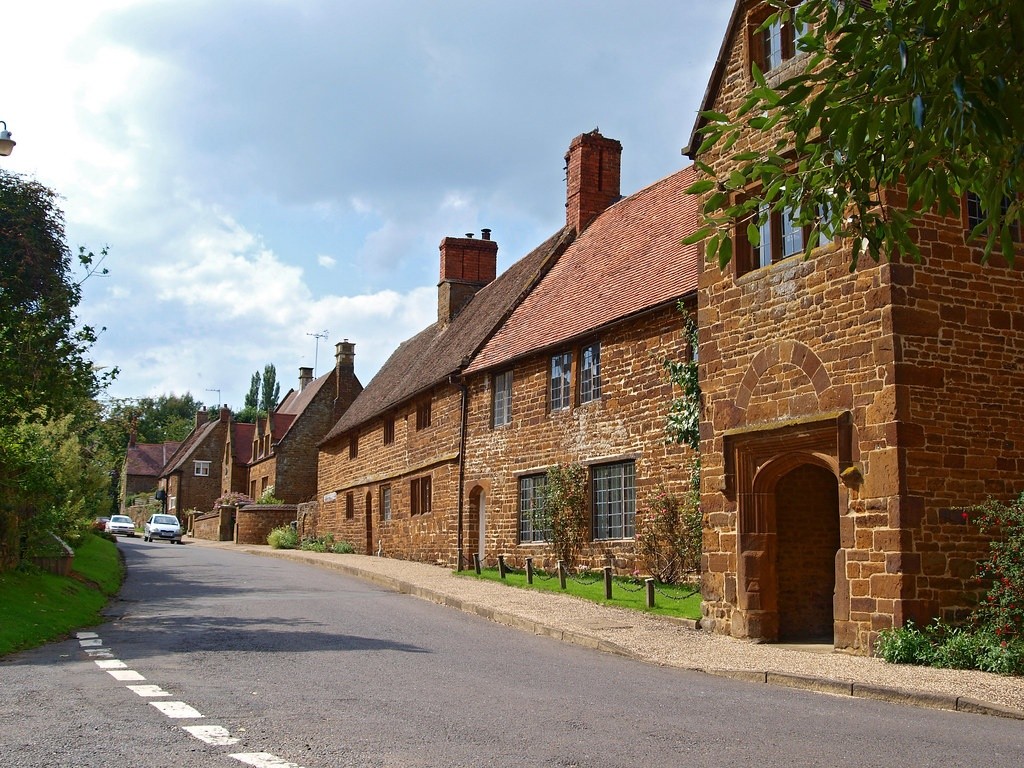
[144,514,181,544]
[96,517,110,529]
[107,515,135,537]
